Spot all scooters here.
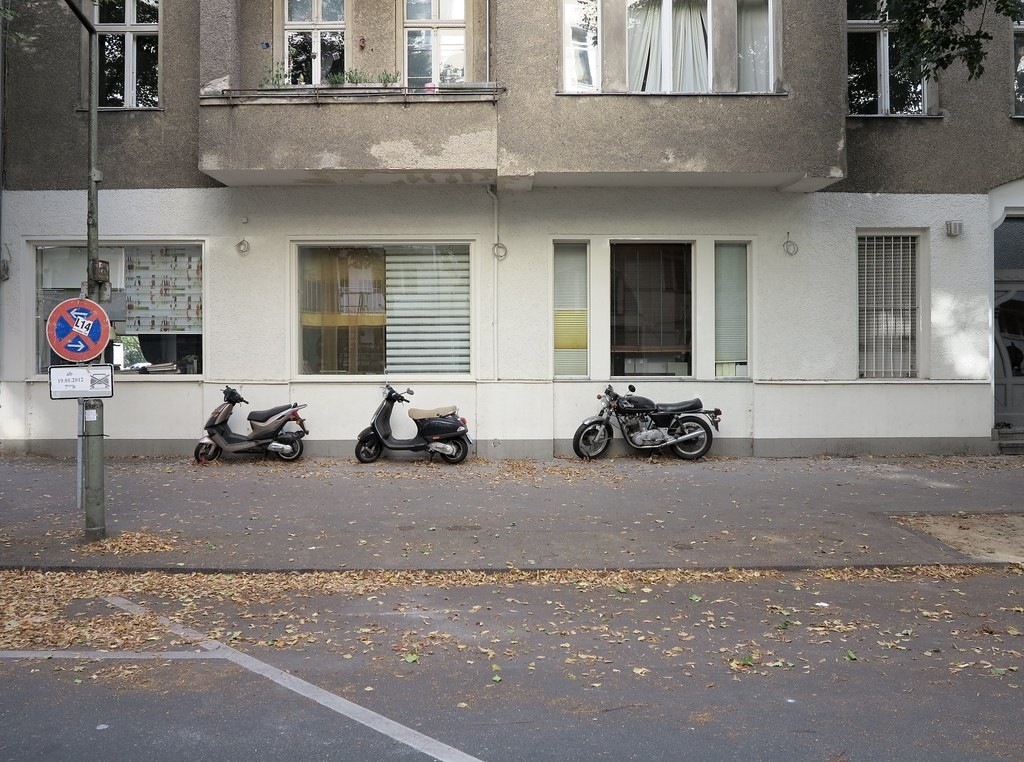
[354,369,474,464]
[193,385,310,464]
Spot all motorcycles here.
[572,384,723,460]
